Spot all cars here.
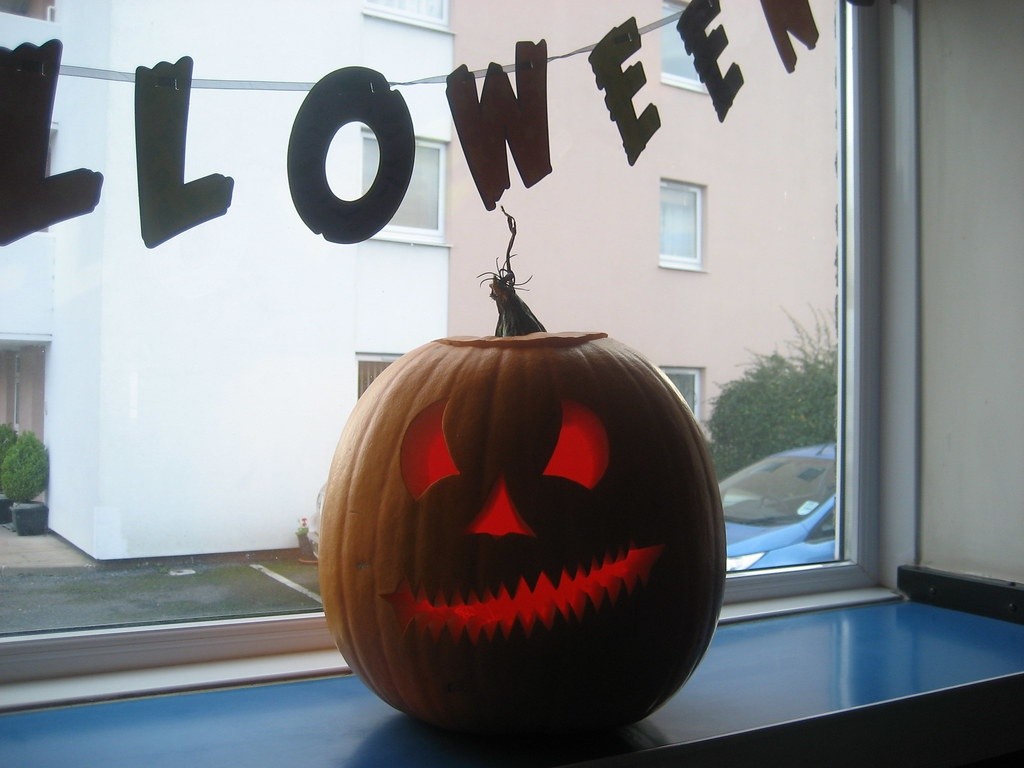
[718,442,839,573]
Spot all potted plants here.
[0,424,50,536]
